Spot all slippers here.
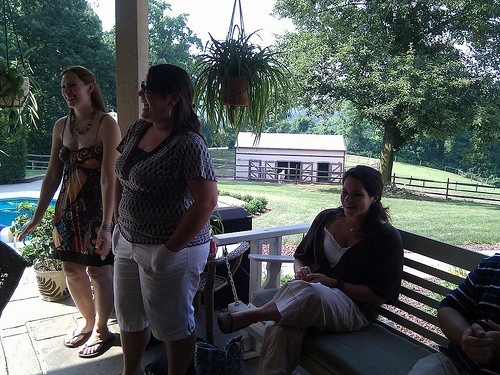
[78,332,113,357]
[62,325,92,348]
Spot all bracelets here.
[101,224,112,232]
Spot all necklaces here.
[350,228,354,231]
[73,109,96,135]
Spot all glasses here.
[141,80,151,94]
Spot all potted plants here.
[0,44,45,144]
[191,24,305,151]
[7,201,70,303]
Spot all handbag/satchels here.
[194,334,244,375]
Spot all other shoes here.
[217,310,257,334]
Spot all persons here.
[20,66,122,358]
[113,63,217,375]
[407,253,500,375]
[217,166,404,375]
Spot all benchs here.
[250,228,490,375]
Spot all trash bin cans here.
[196,194,253,312]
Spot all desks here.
[195,241,250,345]
